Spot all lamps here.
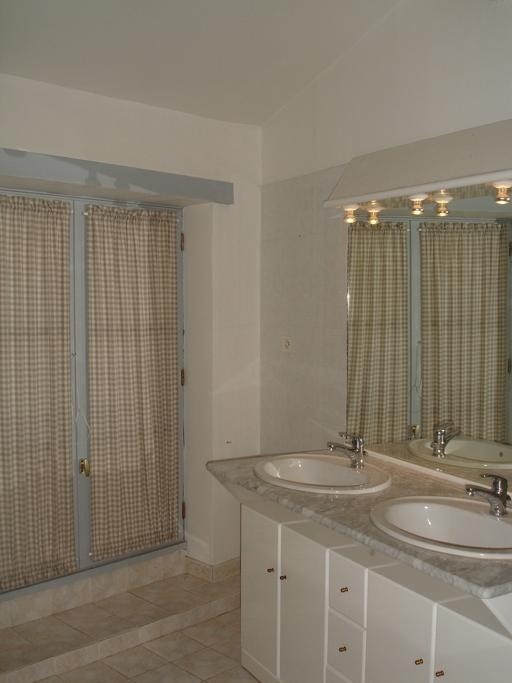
[341,203,359,223]
[491,179,512,204]
[408,192,428,215]
[432,188,454,216]
[366,199,384,224]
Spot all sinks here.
[254,453,393,493]
[369,496,512,560]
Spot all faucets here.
[432,426,463,459]
[463,482,505,516]
[408,435,512,469]
[325,440,357,464]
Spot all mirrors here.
[347,179,512,499]
